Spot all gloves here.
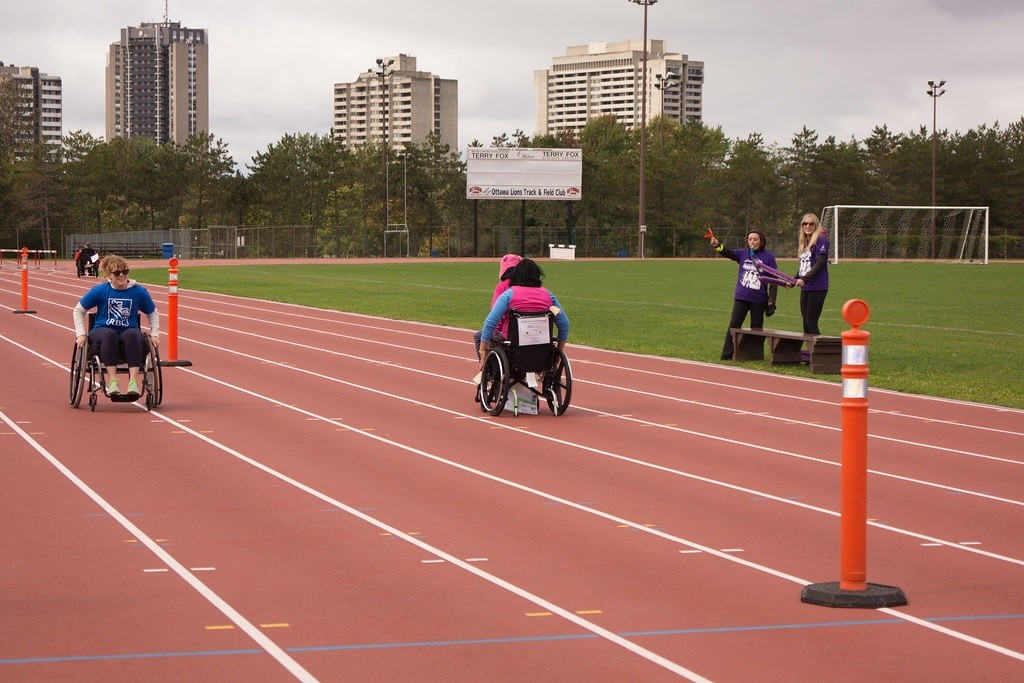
[766,303,776,317]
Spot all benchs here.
[727,326,843,375]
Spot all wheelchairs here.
[69,329,171,411]
[478,346,578,416]
[77,259,100,279]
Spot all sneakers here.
[127,380,139,394]
[107,379,120,395]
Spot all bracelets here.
[478,347,489,354]
[712,243,723,252]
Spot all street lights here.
[926,80,947,256]
[631,1,661,255]
[376,58,394,257]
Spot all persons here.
[784,213,831,363]
[710,229,778,360]
[473,253,569,398]
[74,241,100,277]
[73,255,161,395]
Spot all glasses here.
[802,222,815,226]
[112,269,129,276]
